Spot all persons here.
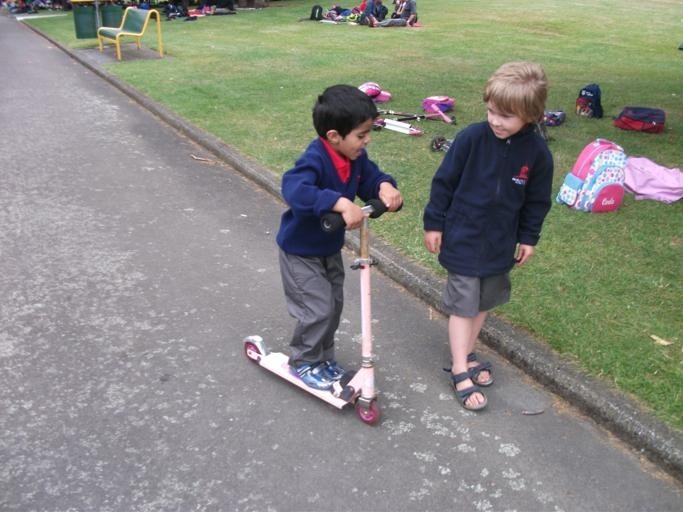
[422,63,552,409]
[366,0,417,28]
[359,0,387,27]
[272,83,402,390]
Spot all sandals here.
[464,351,496,388]
[443,366,488,412]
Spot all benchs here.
[96,6,165,61]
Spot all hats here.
[356,82,391,103]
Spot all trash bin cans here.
[70,0,123,38]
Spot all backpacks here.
[422,94,457,114]
[574,81,606,121]
[542,110,567,127]
[554,137,627,214]
[614,103,668,134]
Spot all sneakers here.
[287,357,347,392]
